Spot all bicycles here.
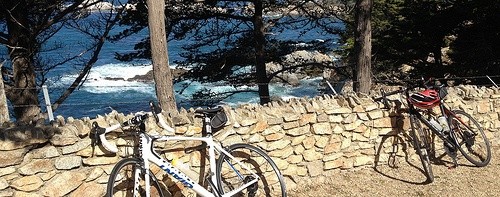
[375,71,492,184]
[91,100,288,197]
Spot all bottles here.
[437,115,451,134]
[171,158,200,180]
[428,116,443,132]
